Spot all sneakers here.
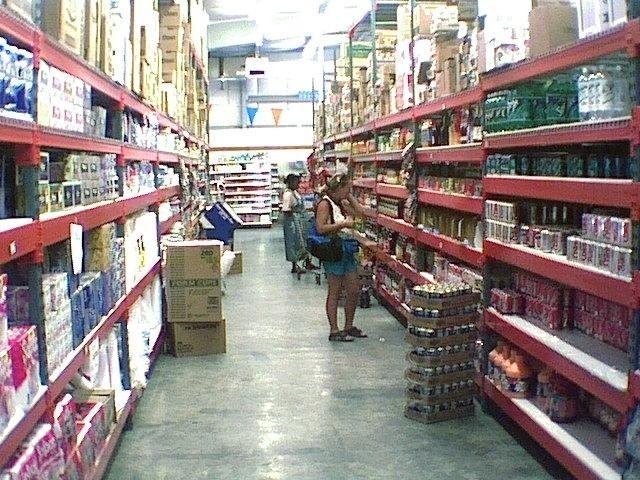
[306,262,315,269]
[291,266,306,273]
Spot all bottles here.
[364,217,418,268]
[376,160,405,187]
[418,206,482,251]
[376,262,401,298]
[418,173,482,198]
[377,196,404,219]
[417,104,484,148]
[482,64,636,134]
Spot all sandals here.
[344,327,367,337]
[328,331,353,342]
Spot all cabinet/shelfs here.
[271,167,280,220]
[0,10,211,480]
[478,18,640,480]
[314,86,483,401]
[211,161,272,226]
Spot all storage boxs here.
[166,320,226,358]
[309,0,477,143]
[0,0,131,145]
[222,250,243,274]
[131,0,212,143]
[165,238,222,322]
[529,4,578,57]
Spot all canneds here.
[489,269,628,352]
[404,280,477,422]
[485,199,633,277]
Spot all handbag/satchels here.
[345,239,358,252]
[304,227,344,262]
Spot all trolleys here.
[301,206,333,284]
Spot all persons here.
[314,172,377,341]
[282,174,317,273]
